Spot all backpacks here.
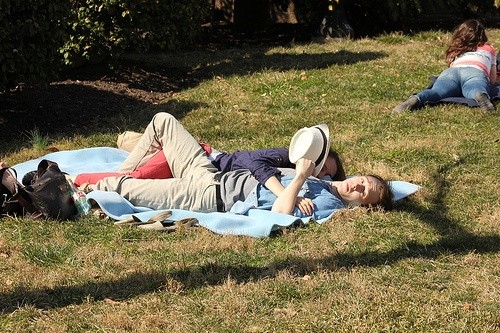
[22,159,91,220]
[0,166,37,219]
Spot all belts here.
[214,172,225,212]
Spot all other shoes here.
[392,95,418,120]
[476,91,495,111]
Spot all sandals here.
[138,218,199,233]
[113,210,172,225]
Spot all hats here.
[288,123,330,176]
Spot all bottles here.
[67,178,92,217]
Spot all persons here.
[391,19,497,113]
[0,113,394,220]
[62,142,346,216]
